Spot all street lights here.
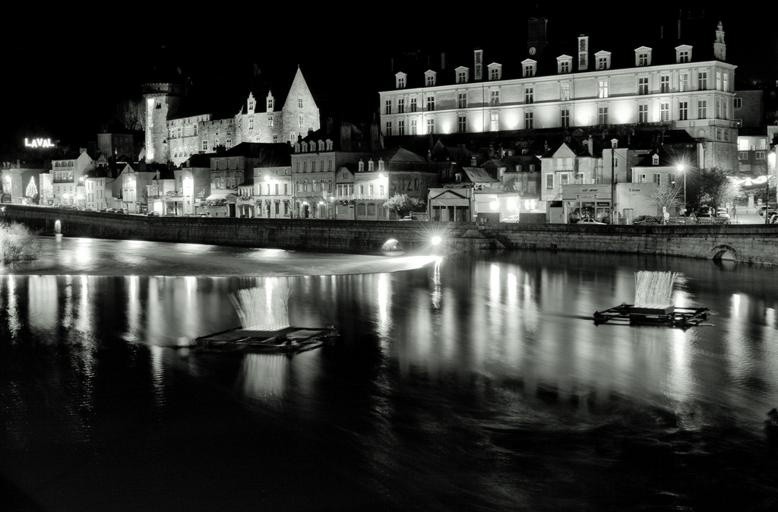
[677,164,688,214]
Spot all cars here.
[758,205,778,224]
[699,205,730,221]
[100,207,126,215]
[578,217,607,225]
